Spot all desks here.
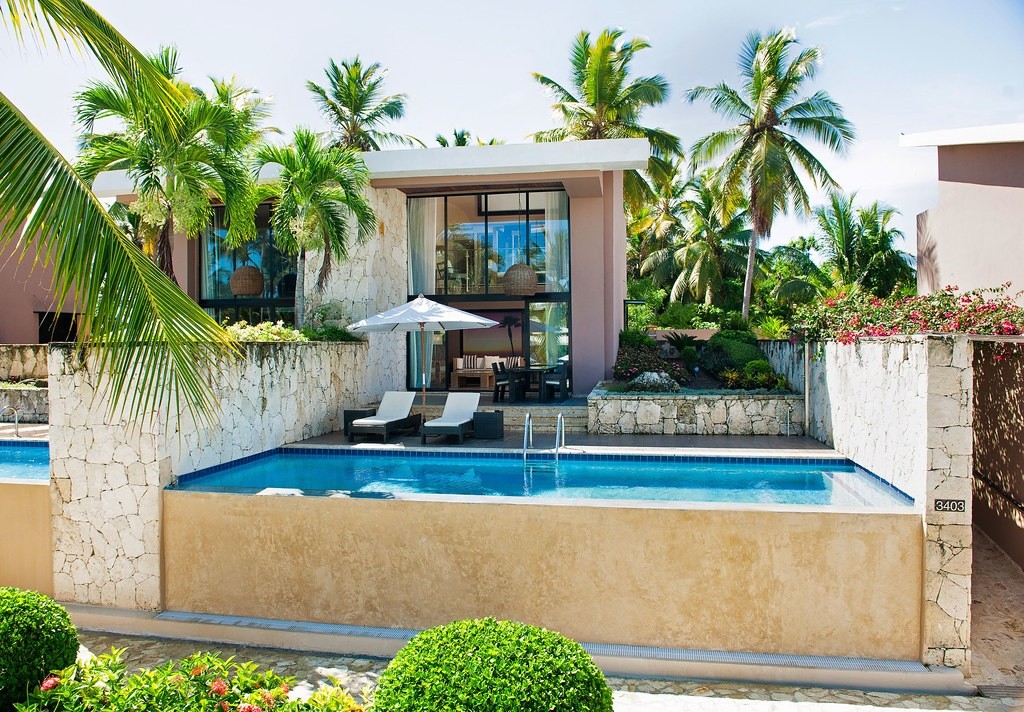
[451,371,495,387]
[508,366,554,402]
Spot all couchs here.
[453,355,525,386]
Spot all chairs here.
[499,362,524,401]
[540,362,570,402]
[349,390,422,443]
[492,363,521,403]
[419,392,482,444]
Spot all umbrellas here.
[346,294,500,423]
[530,319,561,332]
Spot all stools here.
[343,408,376,440]
[473,409,504,440]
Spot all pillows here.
[506,356,520,368]
[484,355,499,369]
[463,355,477,369]
